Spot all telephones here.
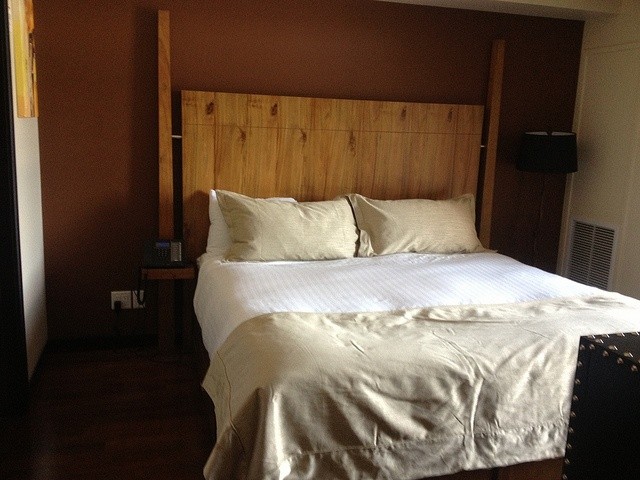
[154,240,185,268]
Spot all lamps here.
[518,130,578,270]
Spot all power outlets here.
[111,290,132,310]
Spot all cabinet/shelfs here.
[142,266,195,282]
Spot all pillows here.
[346,191,496,258]
[215,188,359,263]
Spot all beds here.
[180,89,640,480]
